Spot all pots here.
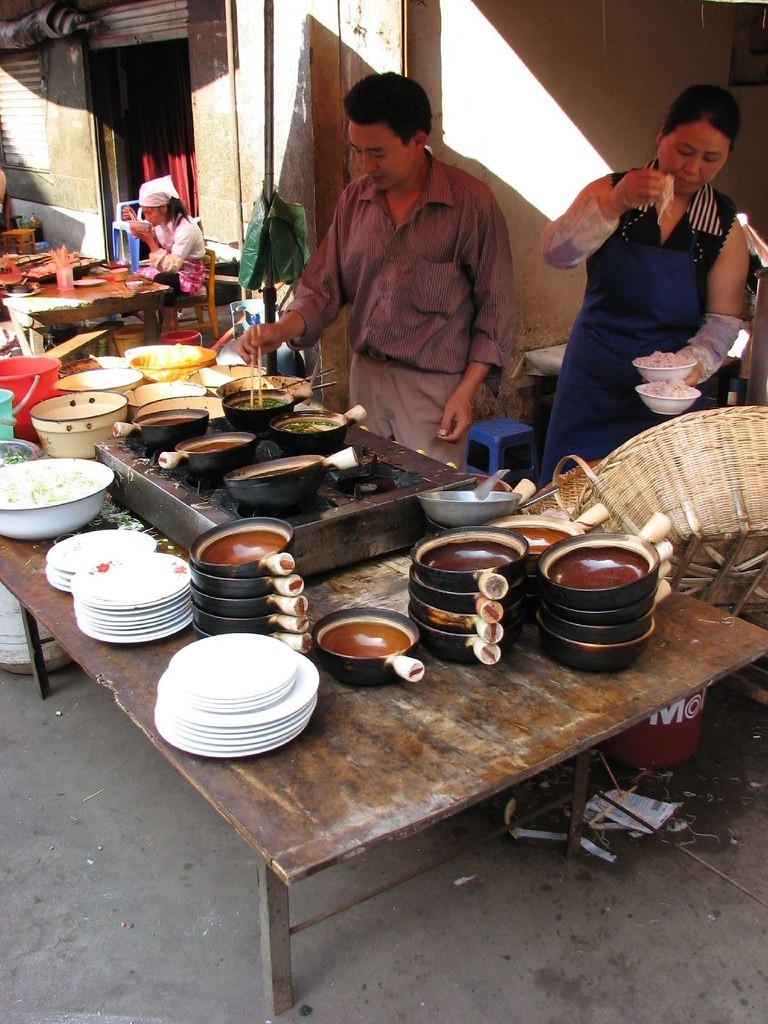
[187,517,313,654]
[113,386,376,512]
[416,469,522,527]
[309,607,426,688]
[406,504,675,673]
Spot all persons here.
[237,72,515,475]
[123,175,206,337]
[540,84,748,492]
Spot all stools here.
[0,229,37,257]
[465,417,539,487]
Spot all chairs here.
[174,248,218,339]
[3,192,12,231]
[111,200,146,274]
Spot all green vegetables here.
[232,399,286,410]
[0,447,26,466]
[280,421,338,433]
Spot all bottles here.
[19,214,32,241]
[30,212,44,243]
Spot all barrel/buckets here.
[113,323,147,357]
[161,329,203,347]
[0,389,18,440]
[0,354,63,444]
[608,685,707,767]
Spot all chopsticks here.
[48,245,69,269]
[128,206,136,221]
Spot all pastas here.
[637,174,674,225]
[642,378,699,398]
[633,350,693,368]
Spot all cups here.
[56,268,75,290]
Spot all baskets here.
[553,406,768,617]
[515,459,603,516]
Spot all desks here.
[0,263,173,357]
[0,491,768,1018]
[510,342,742,448]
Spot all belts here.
[362,347,420,372]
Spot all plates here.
[72,279,107,286]
[154,633,321,760]
[44,529,193,644]
[2,290,39,297]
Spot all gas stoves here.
[94,413,478,578]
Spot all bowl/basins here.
[186,364,267,398]
[126,219,151,231]
[631,358,698,384]
[0,457,115,542]
[216,374,312,396]
[123,344,217,382]
[122,381,207,421]
[134,395,225,419]
[635,382,702,415]
[0,437,40,468]
[27,390,129,459]
[52,367,147,395]
[109,267,128,281]
[5,283,35,293]
[126,281,144,290]
